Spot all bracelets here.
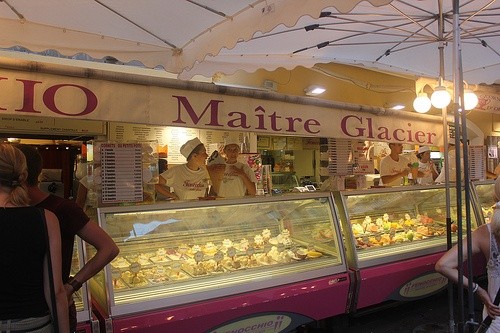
[473,283,478,293]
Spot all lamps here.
[304,83,326,97]
[413,76,479,114]
[384,102,405,111]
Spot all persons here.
[148,135,258,201]
[16,144,120,333]
[70,151,153,213]
[379,143,440,188]
[434,175,500,333]
[0,143,70,333]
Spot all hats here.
[180,137,202,158]
[418,145,430,154]
[224,137,241,151]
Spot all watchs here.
[68,277,83,292]
